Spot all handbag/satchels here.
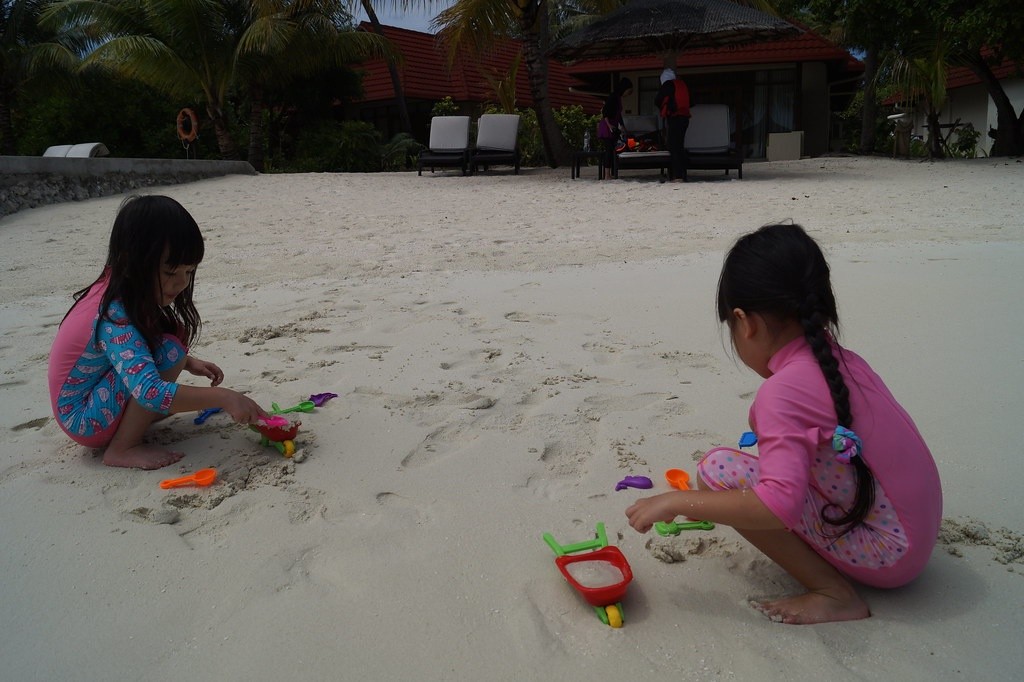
[659,98,677,117]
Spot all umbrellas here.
[543,0,806,174]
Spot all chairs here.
[682,104,744,178]
[469,113,523,177]
[612,114,671,179]
[416,115,475,176]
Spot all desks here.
[569,150,607,180]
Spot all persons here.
[625,220,943,625]
[654,67,691,182]
[47,196,271,468]
[596,78,634,180]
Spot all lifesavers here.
[175,107,198,143]
